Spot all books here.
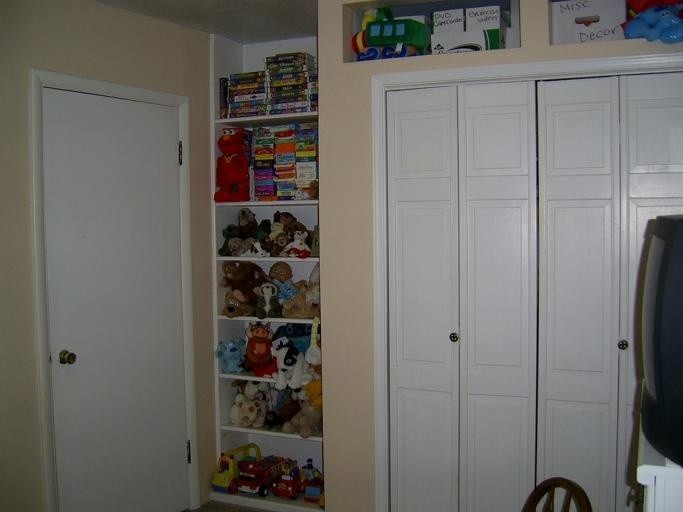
[250,121,317,202]
[218,51,318,119]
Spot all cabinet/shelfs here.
[371,52,682,511]
[209,33,323,511]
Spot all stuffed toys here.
[626,5,683,44]
[212,320,323,438]
[218,208,319,258]
[214,260,320,323]
[214,127,249,204]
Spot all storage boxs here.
[551,1,627,45]
[431,30,500,53]
[431,9,464,33]
[466,6,502,31]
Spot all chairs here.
[521,477,591,510]
